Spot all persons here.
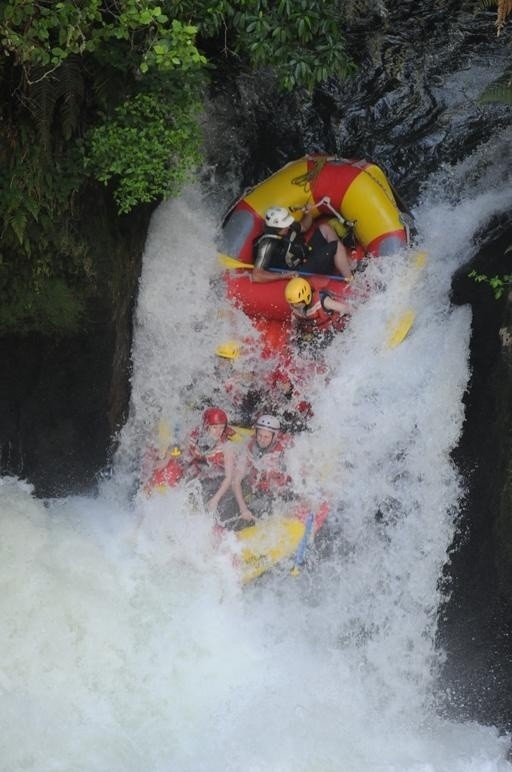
[142,339,328,546]
[284,277,355,359]
[250,206,355,283]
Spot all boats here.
[134,150,428,587]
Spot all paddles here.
[217,250,352,282]
[387,253,425,324]
[390,310,414,346]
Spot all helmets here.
[284,277,312,305]
[201,408,227,430]
[265,205,295,228]
[252,415,280,434]
